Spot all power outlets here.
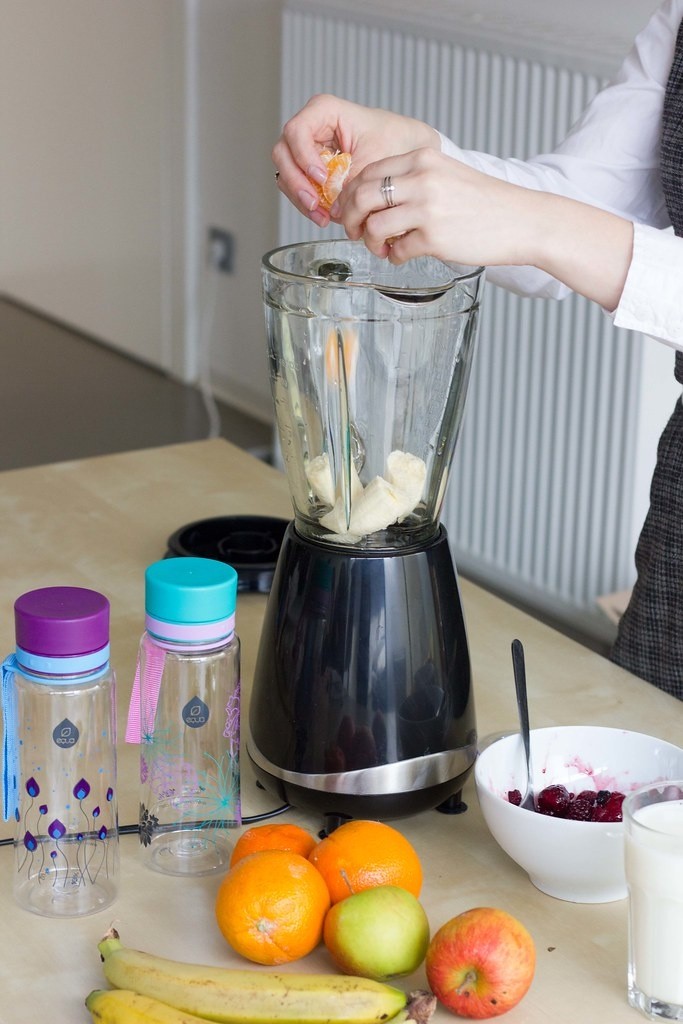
[207,222,235,274]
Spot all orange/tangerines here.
[324,327,353,388]
[216,819,422,964]
[304,148,352,213]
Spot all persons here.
[270,1,683,703]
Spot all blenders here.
[245,240,486,840]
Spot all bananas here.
[84,921,438,1024]
[304,448,427,543]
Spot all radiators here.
[275,0,683,643]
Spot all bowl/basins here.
[475,724,683,904]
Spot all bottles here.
[125,557,242,880]
[0,585,117,918]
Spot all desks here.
[0,439,683,1024]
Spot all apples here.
[426,906,537,1019]
[324,870,431,982]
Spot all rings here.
[378,176,396,207]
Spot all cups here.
[621,779,683,1024]
